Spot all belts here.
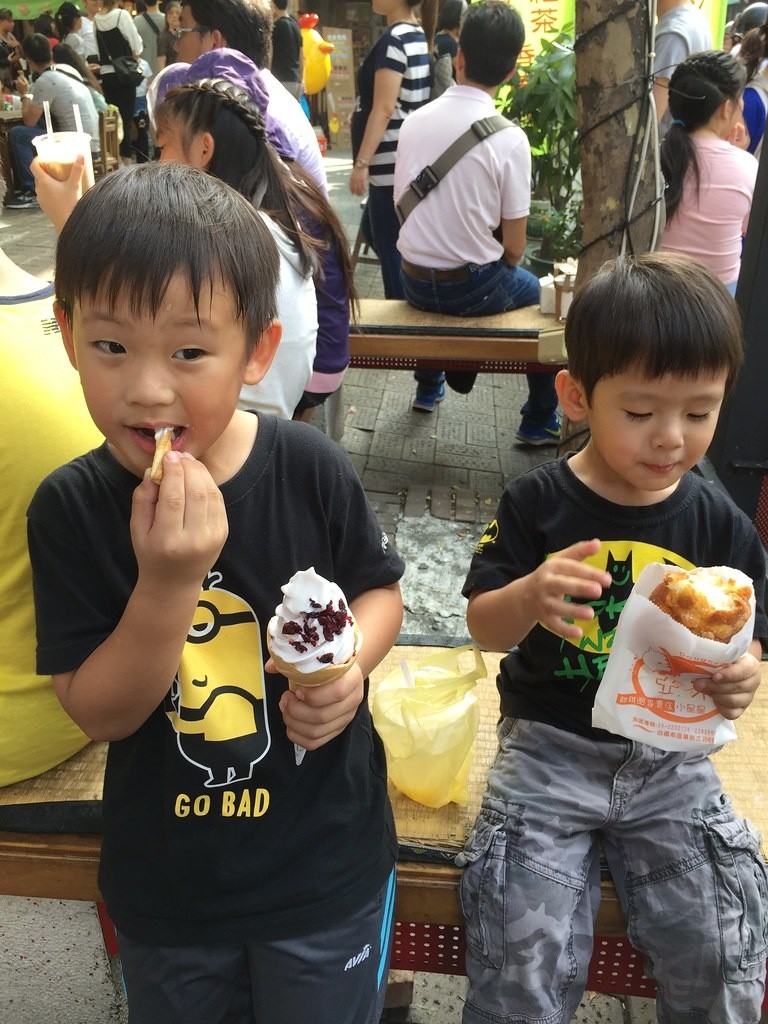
[401,257,472,283]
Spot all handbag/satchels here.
[113,55,145,86]
[371,642,488,810]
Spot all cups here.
[377,664,477,802]
[24,94,34,101]
[12,95,21,109]
[555,275,575,318]
[31,130,95,196]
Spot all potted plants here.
[494,19,584,279]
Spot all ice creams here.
[267,567,363,693]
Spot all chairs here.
[92,110,120,183]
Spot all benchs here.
[0,634,768,1024]
[324,299,569,444]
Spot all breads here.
[648,570,753,645]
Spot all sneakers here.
[413,384,445,412]
[514,409,565,444]
[5,189,40,208]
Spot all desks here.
[0,110,24,192]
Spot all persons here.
[28,162,406,1024]
[458,252,768,1024]
[0,250,111,793]
[155,79,321,422]
[0,0,768,302]
[160,46,360,414]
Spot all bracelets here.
[20,94,30,102]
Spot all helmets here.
[729,2,768,39]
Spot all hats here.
[59,1,89,17]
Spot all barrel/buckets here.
[318,139,327,157]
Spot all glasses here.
[174,26,213,40]
